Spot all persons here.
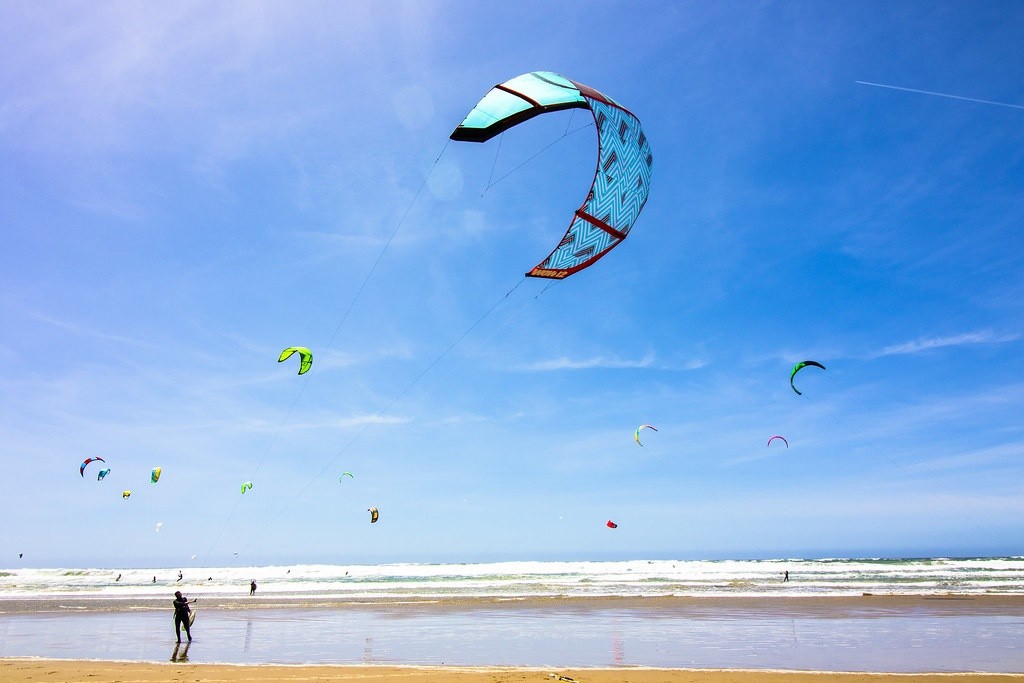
[173,591,197,644]
[250,580,256,595]
[176,570,183,582]
[153,576,156,583]
[784,571,788,582]
[116,574,121,581]
